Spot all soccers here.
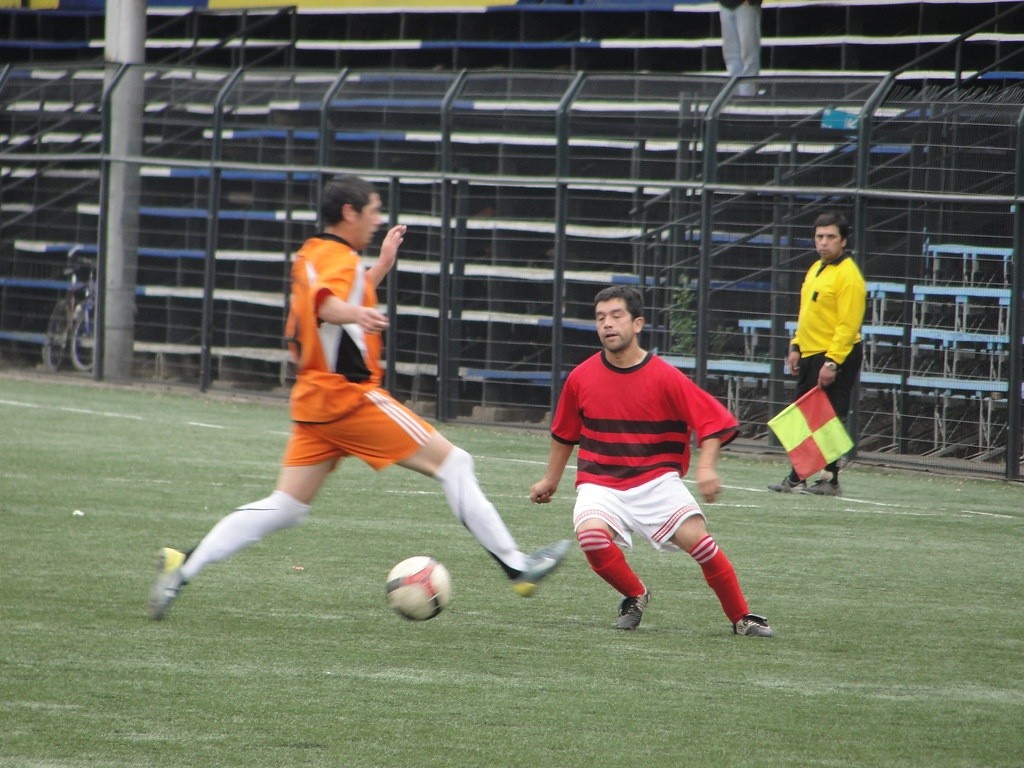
[385,557,451,620]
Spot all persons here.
[768,213,867,496]
[149,177,570,622]
[718,0,762,98]
[529,287,773,639]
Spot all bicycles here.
[42,243,99,373]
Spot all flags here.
[767,385,854,481]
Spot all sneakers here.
[148,547,189,620]
[800,479,842,496]
[512,539,571,597]
[614,578,651,630]
[768,476,807,493]
[733,613,773,637]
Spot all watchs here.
[824,363,841,374]
